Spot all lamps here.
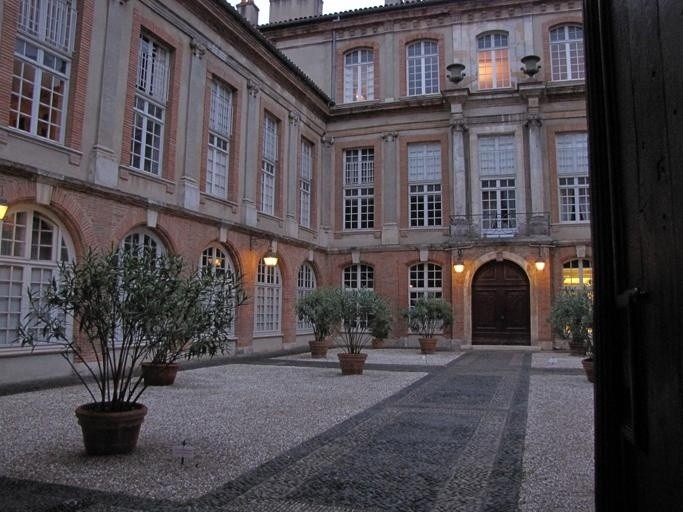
[0,198,8,220]
[208,258,222,267]
[250,233,279,266]
[534,244,546,271]
[454,246,464,273]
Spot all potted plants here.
[371,320,392,348]
[9,240,214,455]
[402,294,454,354]
[142,263,254,386]
[545,274,592,356]
[576,280,594,382]
[293,287,347,358]
[323,284,394,375]
